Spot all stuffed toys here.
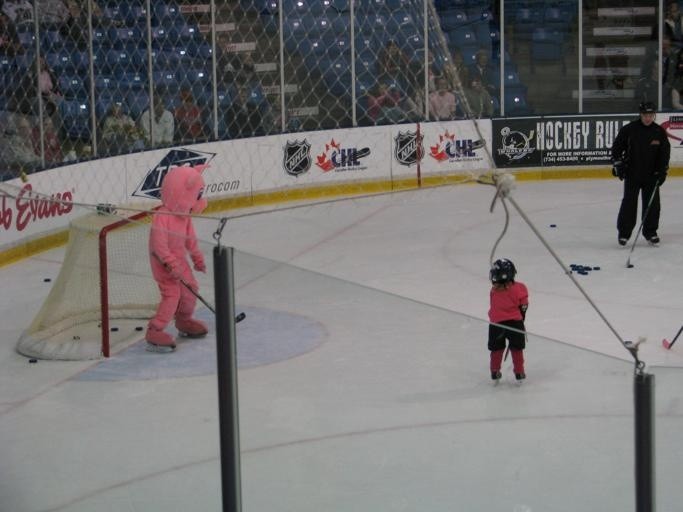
[145,164,207,347]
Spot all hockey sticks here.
[662,325,683,351]
[626,176,659,268]
[148,250,245,325]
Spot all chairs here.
[1,1,270,161]
[261,0,579,132]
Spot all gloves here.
[612,160,626,181]
[655,170,667,186]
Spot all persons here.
[611,102,670,244]
[634,0,683,113]
[488,258,530,380]
[368,42,497,127]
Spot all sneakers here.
[491,372,526,380]
[618,231,631,246]
[642,230,660,243]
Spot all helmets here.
[489,258,516,283]
[639,101,657,114]
